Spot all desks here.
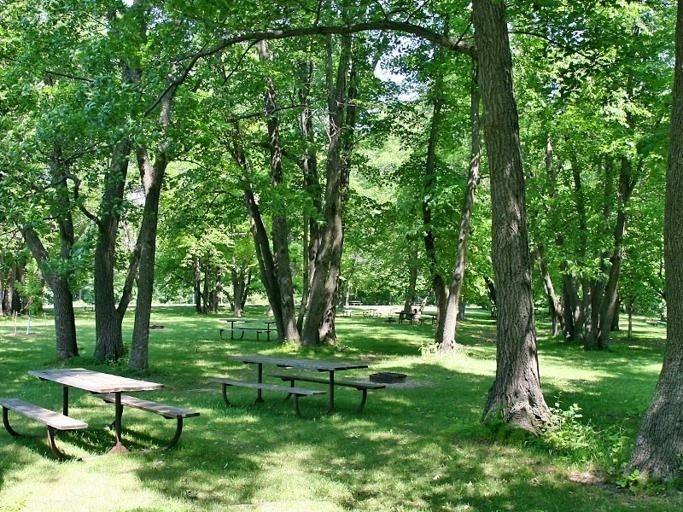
[23,364,172,458]
[231,351,369,417]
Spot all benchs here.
[200,373,330,423]
[268,370,387,417]
[210,295,441,345]
[86,391,201,452]
[0,396,88,465]
